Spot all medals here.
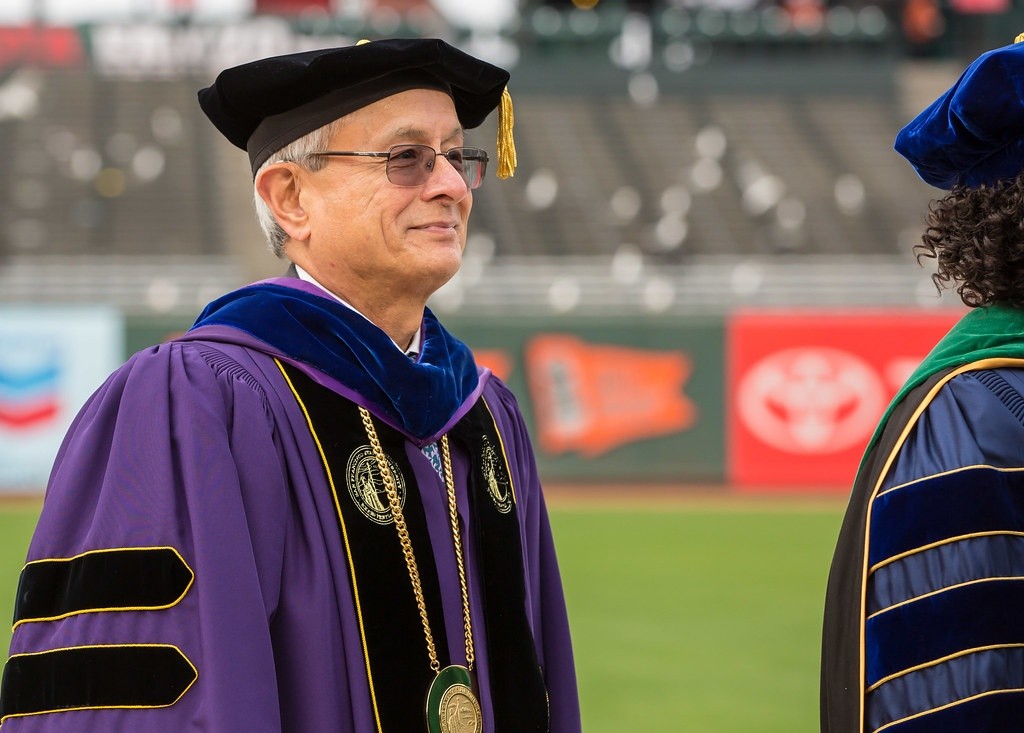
[424,662,483,733]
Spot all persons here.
[819,32,1024,733]
[0,39,582,733]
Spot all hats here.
[197,39,517,178]
[894,42,1024,190]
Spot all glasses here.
[309,145,489,189]
